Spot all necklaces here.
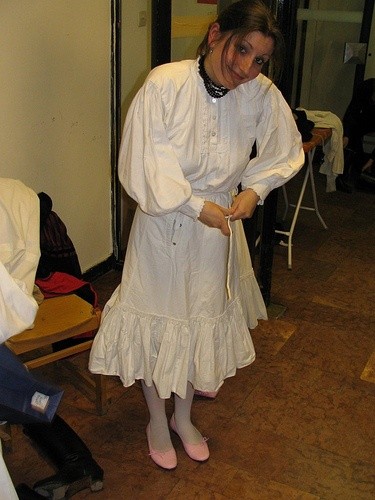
[197,53,230,98]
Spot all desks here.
[250,119,334,270]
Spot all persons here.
[87,0,306,470]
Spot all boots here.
[21,412,105,498]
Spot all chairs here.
[4,191,107,416]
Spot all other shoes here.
[171,412,210,461]
[147,423,178,470]
